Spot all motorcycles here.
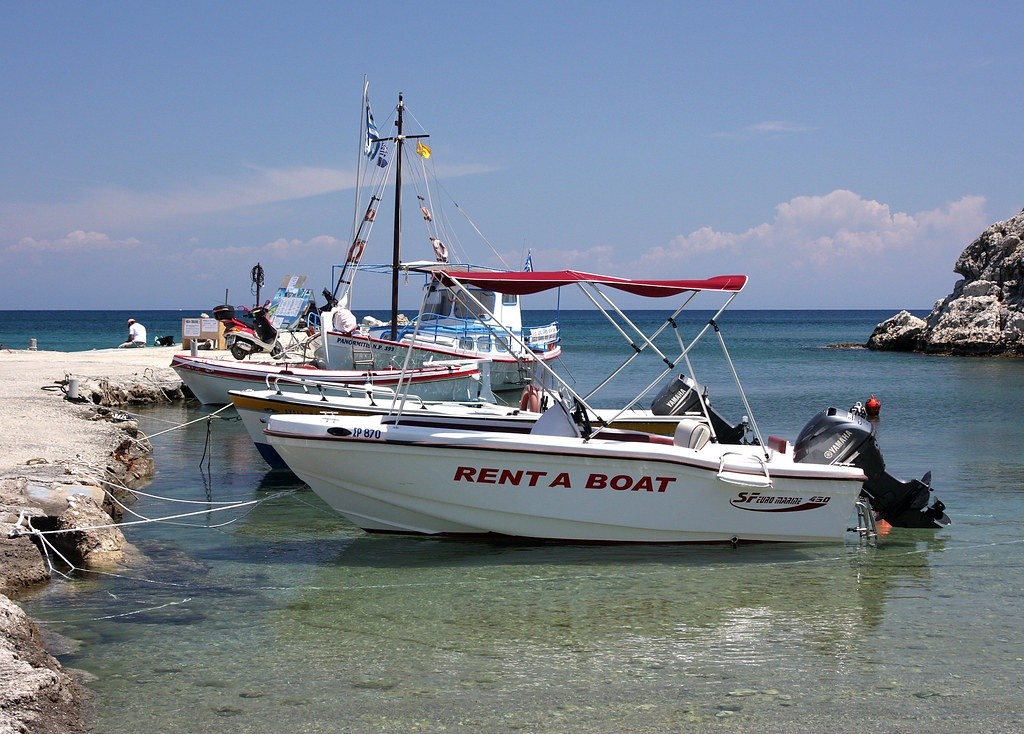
[215,300,285,360]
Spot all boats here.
[171,73,953,546]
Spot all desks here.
[282,328,320,352]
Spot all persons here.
[119,319,147,347]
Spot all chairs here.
[352,334,375,370]
[672,418,712,451]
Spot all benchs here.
[182,318,226,350]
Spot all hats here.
[127,319,135,325]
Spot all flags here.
[416,140,431,158]
[524,253,533,272]
[363,95,388,169]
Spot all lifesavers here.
[348,241,365,263]
[364,208,376,222]
[433,240,449,260]
[421,206,432,221]
